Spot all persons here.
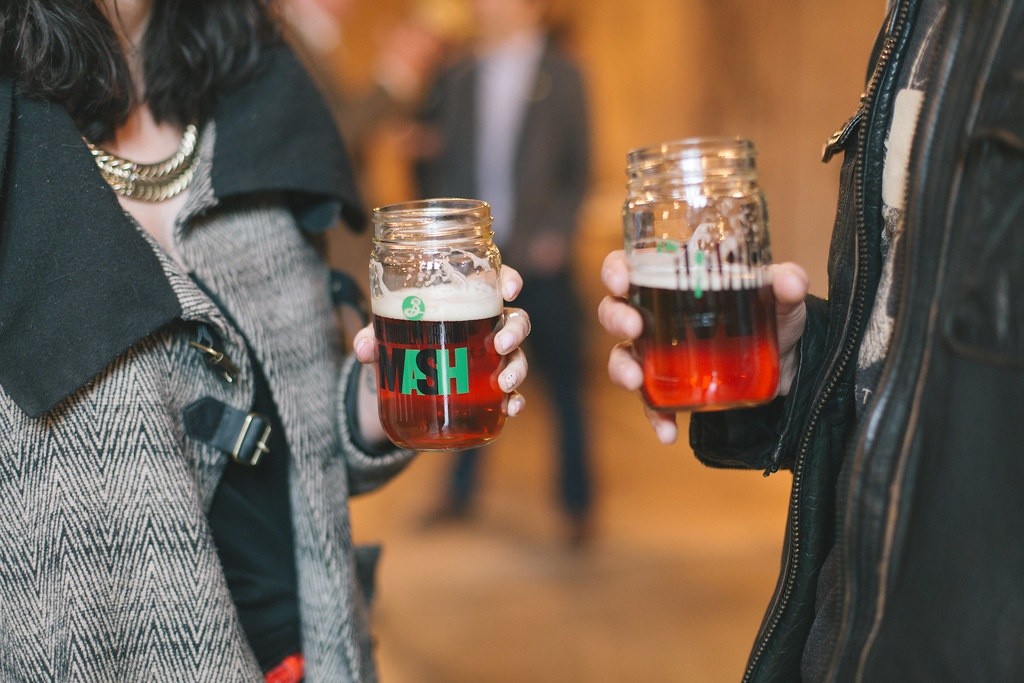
[391,1,599,545]
[598,0,1024,683]
[0,0,532,683]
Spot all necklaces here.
[82,122,207,202]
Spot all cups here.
[621,136,781,414]
[369,197,512,453]
